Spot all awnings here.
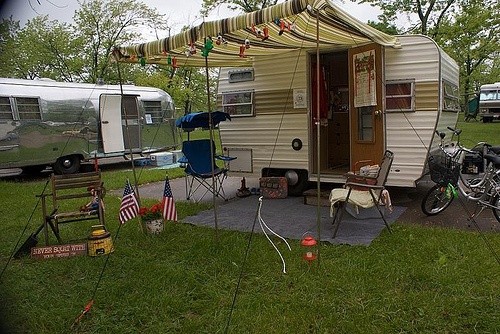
[0,0,500,334]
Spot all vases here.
[140,217,165,238]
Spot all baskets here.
[428,156,460,186]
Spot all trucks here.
[476,82,500,123]
[0,76,177,180]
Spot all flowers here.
[136,202,165,222]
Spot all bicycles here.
[421,126,500,227]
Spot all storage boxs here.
[170,149,184,164]
[149,151,173,167]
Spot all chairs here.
[35,166,105,247]
[330,149,396,240]
[177,138,238,206]
[467,107,480,123]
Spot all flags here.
[161,177,177,221]
[118,178,140,224]
